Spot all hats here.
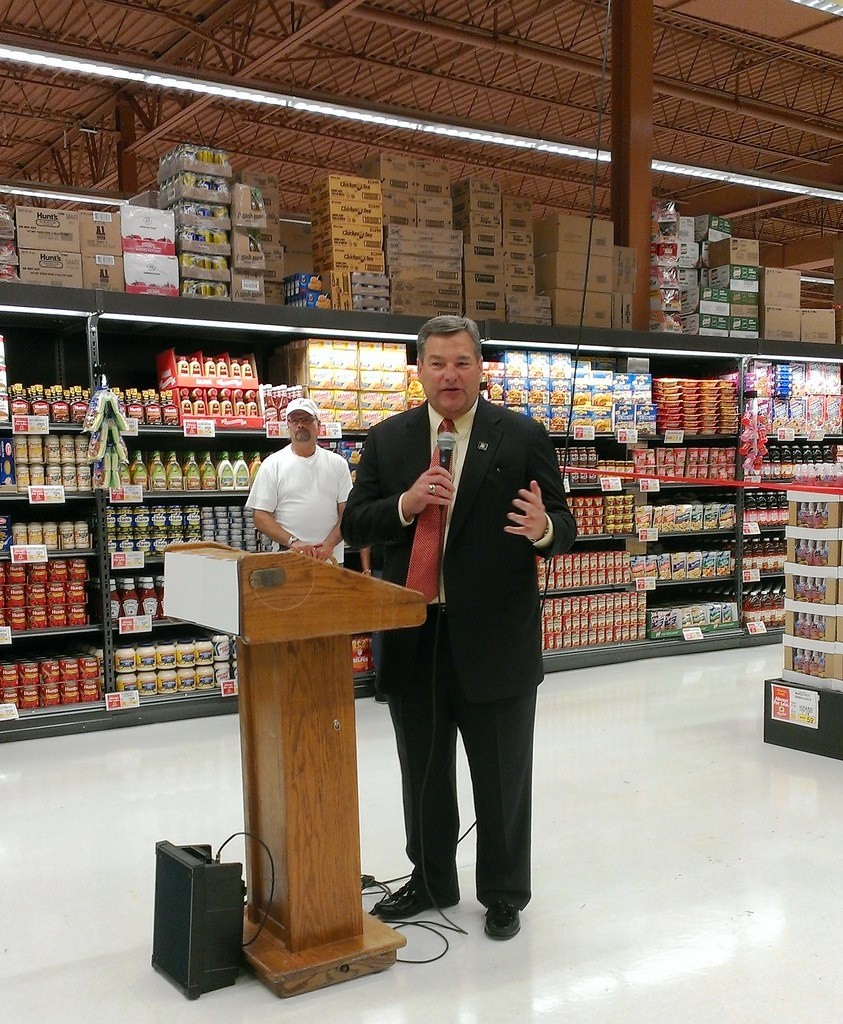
[285,398,318,425]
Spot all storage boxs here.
[750,360,843,435]
[781,484,843,691]
[318,439,365,482]
[128,191,158,208]
[357,153,638,330]
[535,549,738,650]
[14,203,179,296]
[155,160,390,314]
[156,339,427,430]
[479,347,658,434]
[650,199,842,344]
[0,335,13,553]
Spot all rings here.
[428,484,436,494]
[299,550,303,554]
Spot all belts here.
[427,605,448,622]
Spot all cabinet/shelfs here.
[741,336,843,645]
[0,280,111,744]
[475,320,741,673]
[94,289,431,729]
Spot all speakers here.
[151,840,245,1001]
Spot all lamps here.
[1,42,613,164]
[652,160,842,202]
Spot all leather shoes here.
[374,880,460,919]
[485,897,521,941]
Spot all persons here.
[248,397,354,570]
[339,313,579,939]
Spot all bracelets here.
[362,568,371,575]
[526,514,549,543]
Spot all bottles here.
[73,633,231,694]
[688,445,839,679]
[13,434,93,548]
[111,576,171,622]
[8,357,302,425]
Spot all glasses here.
[289,417,318,425]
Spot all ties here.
[406,418,455,602]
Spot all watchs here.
[287,535,299,548]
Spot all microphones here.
[437,432,456,511]
